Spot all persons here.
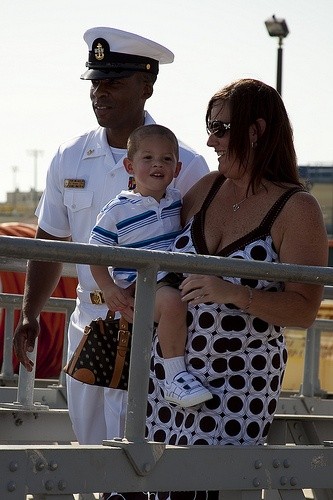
[89,124,213,408]
[119,79,330,500]
[13,42,210,445]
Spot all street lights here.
[266,14,290,98]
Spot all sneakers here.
[164,371,213,407]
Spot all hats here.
[80,27,174,81]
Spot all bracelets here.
[240,284,254,314]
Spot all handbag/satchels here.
[62,306,134,391]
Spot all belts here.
[76,288,105,305]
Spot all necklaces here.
[230,179,268,212]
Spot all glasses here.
[206,121,231,138]
